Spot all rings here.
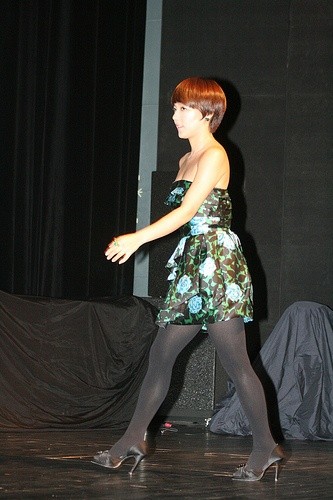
[114,242,120,246]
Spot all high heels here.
[232,443,284,483]
[91,429,150,476]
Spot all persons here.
[92,76,287,484]
